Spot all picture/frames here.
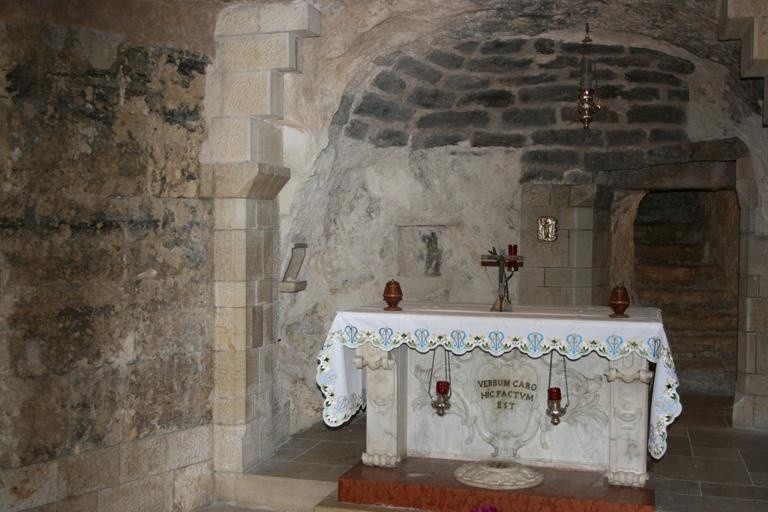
[535,216,559,243]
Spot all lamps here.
[547,349,570,424]
[609,284,630,318]
[575,21,602,132]
[427,346,452,416]
[382,278,403,311]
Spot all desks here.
[321,302,684,512]
